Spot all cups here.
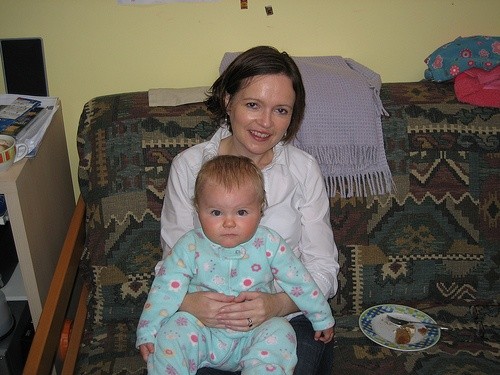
[0,135,28,172]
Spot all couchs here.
[22,81,500,375]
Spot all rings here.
[248,318,253,327]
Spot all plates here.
[358,304,440,351]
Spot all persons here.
[155,46,341,375]
[135,154,336,375]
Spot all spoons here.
[386,314,450,330]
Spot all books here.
[0,104,47,142]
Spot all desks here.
[0,99,77,375]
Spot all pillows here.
[425,34,500,83]
[453,65,500,108]
